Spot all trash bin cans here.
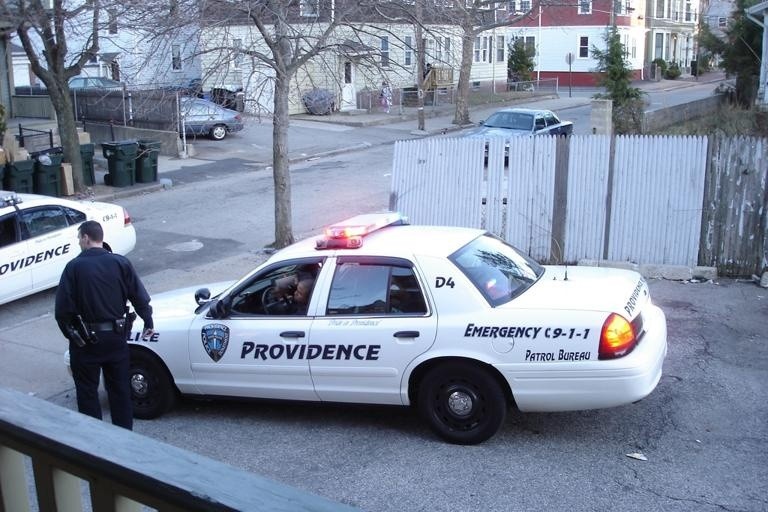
[0,138,162,197]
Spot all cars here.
[60,213,669,445]
[0,191,138,305]
[461,106,575,165]
[128,95,243,141]
[68,77,126,91]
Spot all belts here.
[87,321,114,332]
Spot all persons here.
[270,262,321,315]
[379,82,393,114]
[55,219,156,430]
